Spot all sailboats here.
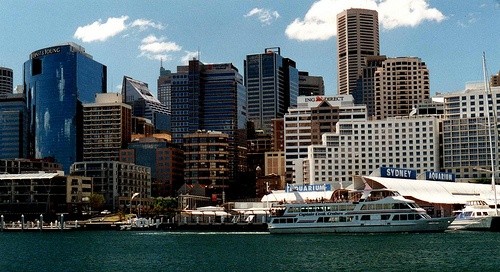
[446,50,500,230]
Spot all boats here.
[267,188,454,233]
